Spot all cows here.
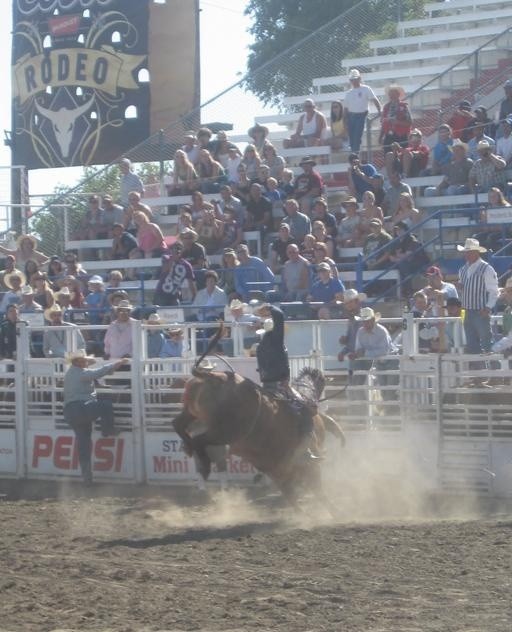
[171,318,347,517]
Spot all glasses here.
[120,310,129,315]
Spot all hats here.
[227,298,247,315]
[44,303,65,320]
[248,123,268,138]
[64,347,96,365]
[142,313,165,324]
[195,358,216,370]
[425,266,440,276]
[472,140,491,151]
[3,270,26,289]
[88,274,104,292]
[384,82,405,97]
[113,299,133,312]
[298,156,316,167]
[341,196,359,210]
[448,138,468,153]
[167,323,183,335]
[349,69,360,79]
[53,287,75,301]
[256,319,288,334]
[442,297,461,308]
[16,233,37,250]
[23,286,32,296]
[457,238,487,253]
[336,289,367,305]
[353,307,381,323]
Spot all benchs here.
[2,0,511,400]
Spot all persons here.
[62,348,129,486]
[256,304,326,462]
[0,67,512,425]
